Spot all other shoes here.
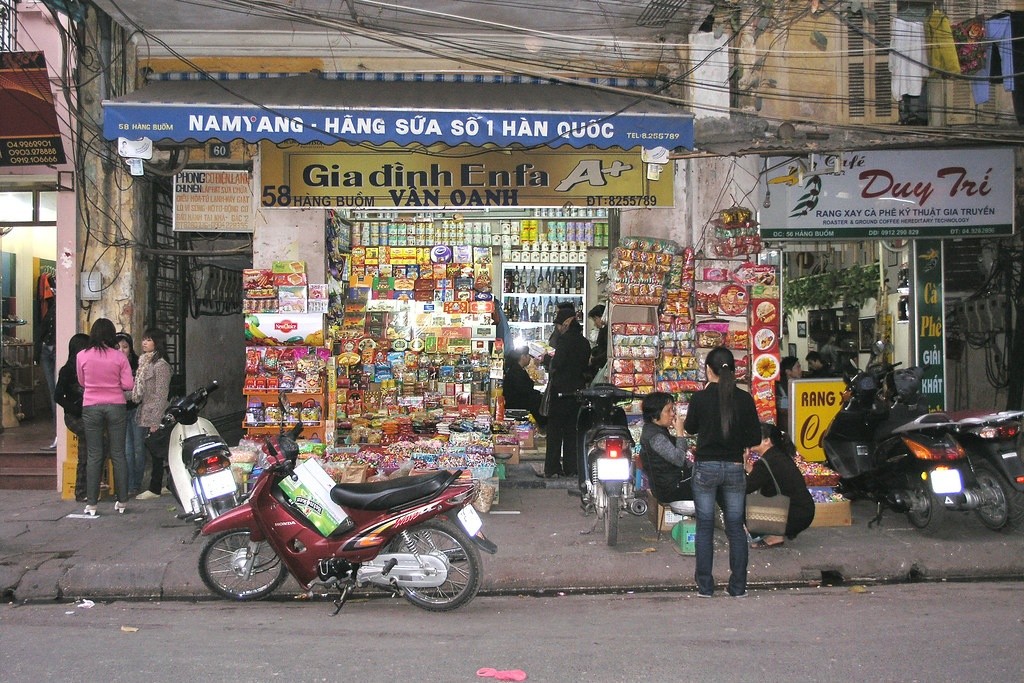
[114,488,139,501]
[696,591,712,597]
[75,495,87,502]
[724,587,749,597]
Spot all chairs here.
[640,439,682,540]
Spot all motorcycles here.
[147,378,245,542]
[821,337,1024,537]
[195,392,497,616]
[558,384,649,547]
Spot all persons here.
[505,302,608,479]
[639,351,857,550]
[33,299,57,448]
[683,345,761,597]
[55,318,175,515]
[2,369,22,429]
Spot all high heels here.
[565,472,578,477]
[545,471,560,478]
[115,501,125,514]
[84,505,96,515]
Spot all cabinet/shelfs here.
[244,349,325,448]
[810,305,860,377]
[694,257,753,395]
[345,210,621,249]
[499,263,586,347]
[3,342,36,420]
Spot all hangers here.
[889,2,923,24]
[42,265,56,277]
[977,0,1011,23]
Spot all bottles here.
[562,297,575,307]
[530,296,542,316]
[564,267,572,294]
[697,292,718,314]
[728,287,738,304]
[504,297,528,322]
[545,296,559,323]
[522,266,527,283]
[530,266,536,284]
[546,267,550,283]
[517,277,525,293]
[575,267,584,294]
[552,266,564,294]
[504,272,512,293]
[417,351,490,384]
[538,267,543,287]
[246,397,319,426]
[513,266,520,293]
[526,278,537,293]
[576,297,583,321]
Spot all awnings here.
[101,74,696,152]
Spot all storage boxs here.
[606,359,656,396]
[812,498,852,527]
[607,304,661,360]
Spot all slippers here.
[161,487,172,495]
[136,490,160,500]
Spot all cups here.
[762,274,774,285]
[737,292,744,299]
[703,330,722,346]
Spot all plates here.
[754,354,779,380]
[756,302,775,323]
[754,329,775,350]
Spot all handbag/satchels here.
[746,458,790,535]
[145,429,169,458]
[539,358,556,417]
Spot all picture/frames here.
[788,342,797,359]
[797,321,806,338]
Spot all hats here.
[553,308,576,324]
